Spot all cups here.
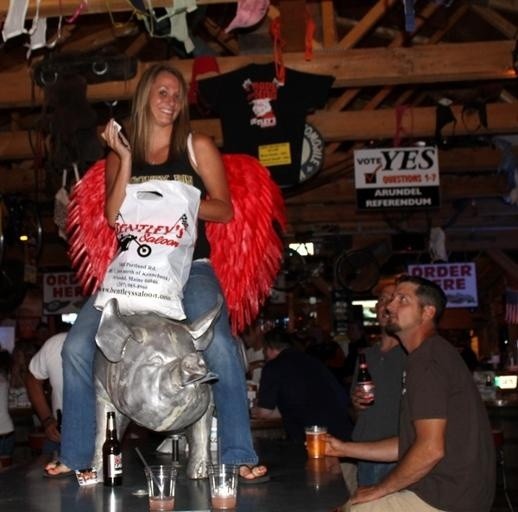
[144,465,177,512]
[207,464,240,512]
[306,425,328,459]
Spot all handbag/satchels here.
[92,179,201,320]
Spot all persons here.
[12,319,48,387]
[24,330,71,454]
[44,60,273,484]
[242,269,496,511]
[1,342,16,436]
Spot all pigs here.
[92,298,225,481]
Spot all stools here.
[492,429,514,512]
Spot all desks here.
[0,418,351,512]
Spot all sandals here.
[40,458,93,479]
[238,463,271,484]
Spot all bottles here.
[55,409,62,456]
[358,352,376,406]
[102,411,124,486]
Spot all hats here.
[374,272,412,293]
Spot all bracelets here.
[41,416,56,430]
